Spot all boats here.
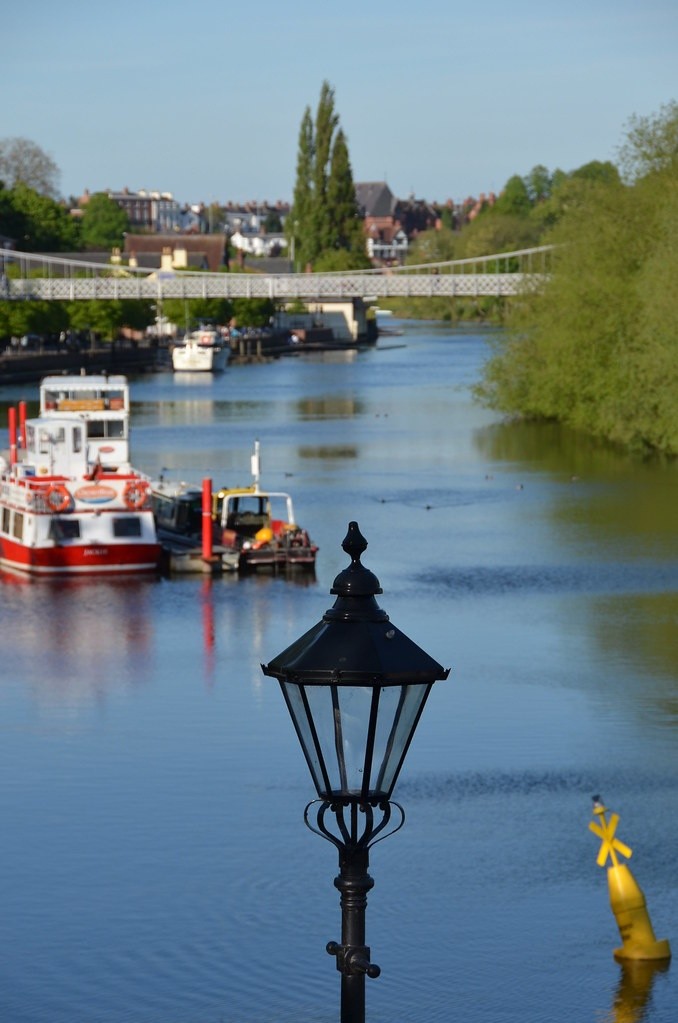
[144,438,320,580]
[0,368,160,587]
[168,329,232,372]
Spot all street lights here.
[258,519,453,1023]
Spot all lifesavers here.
[122,482,148,508]
[42,485,72,513]
